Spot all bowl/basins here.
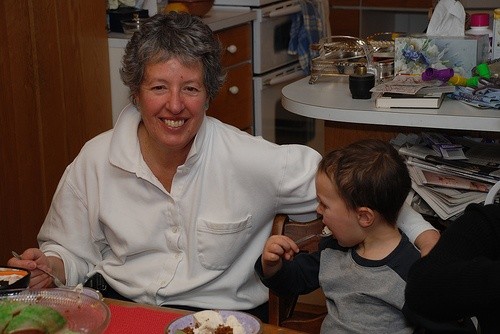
[167,0,215,17]
[107,9,148,33]
[0,266,31,296]
[121,18,147,35]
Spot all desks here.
[281,75,500,159]
[43,287,305,334]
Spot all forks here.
[11,250,65,288]
[294,226,333,244]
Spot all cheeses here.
[322,226,333,237]
[191,311,246,334]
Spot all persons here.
[7,10,440,323]
[253,137,422,334]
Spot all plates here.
[0,288,111,334]
[65,286,103,302]
[164,309,262,334]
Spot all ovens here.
[213,0,324,155]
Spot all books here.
[375,92,444,110]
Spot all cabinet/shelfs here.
[108,21,254,135]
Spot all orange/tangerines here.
[164,3,190,15]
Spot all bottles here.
[464,13,492,61]
